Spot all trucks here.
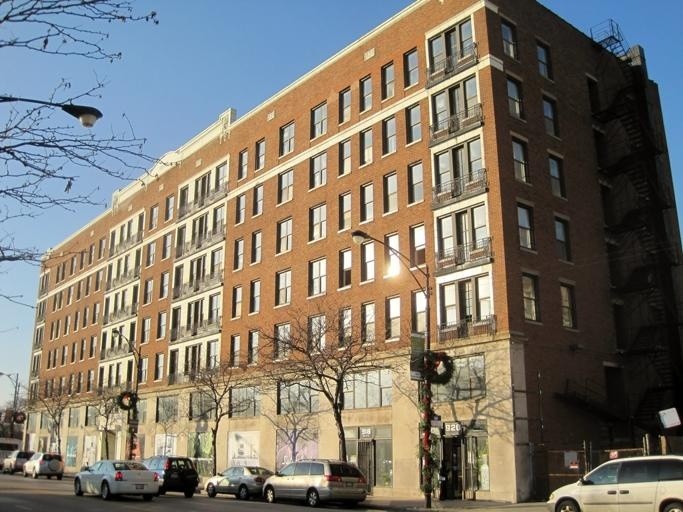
[0,436,24,473]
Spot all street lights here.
[2,94,106,130]
[0,371,23,438]
[345,226,440,505]
[110,327,143,459]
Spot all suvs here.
[3,450,34,476]
[545,454,683,512]
[22,452,67,481]
[261,456,370,507]
[141,455,201,500]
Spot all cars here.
[71,460,162,501]
[201,465,272,498]
